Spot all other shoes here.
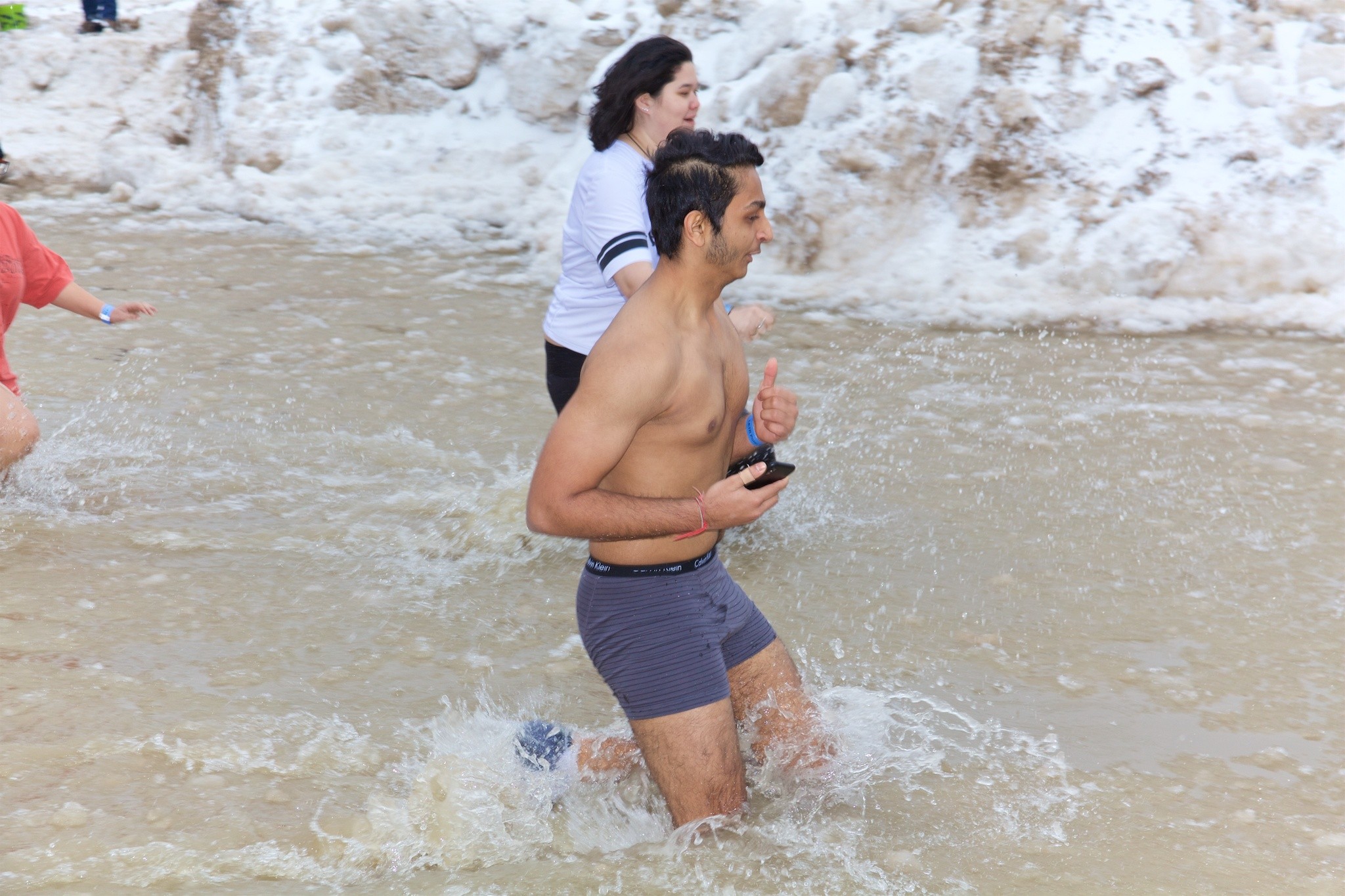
[81,19,115,33]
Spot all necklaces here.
[627,132,650,158]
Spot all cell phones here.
[746,461,796,489]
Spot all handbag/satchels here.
[0,3,28,31]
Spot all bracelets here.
[745,414,764,446]
[674,485,707,542]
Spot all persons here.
[0,139,157,474]
[515,126,836,833]
[77,0,116,33]
[543,36,699,412]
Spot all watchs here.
[99,304,115,324]
